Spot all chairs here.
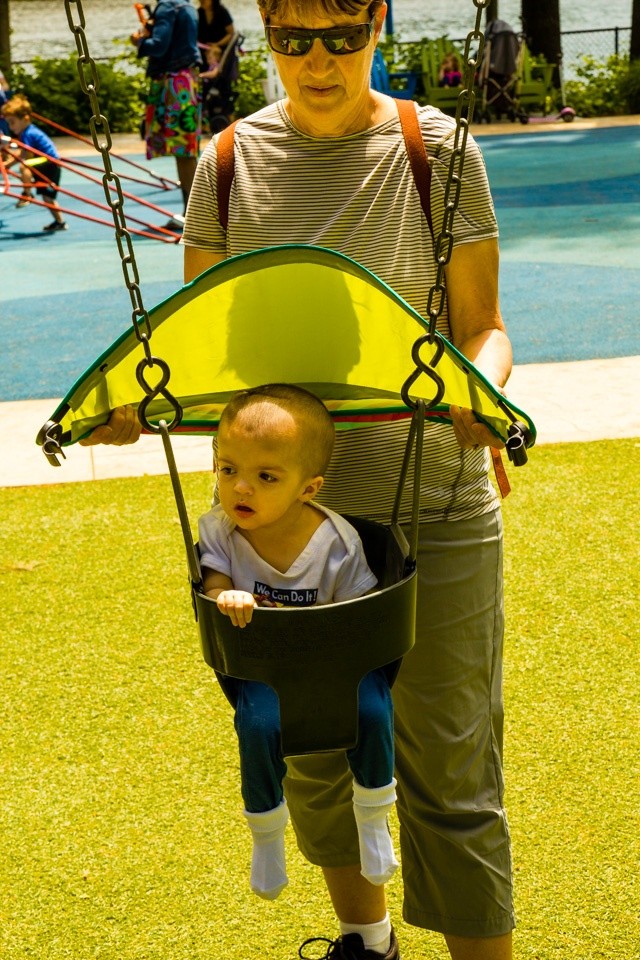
[370,37,557,121]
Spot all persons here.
[72,5,515,960]
[438,53,462,86]
[196,385,399,902]
[128,0,242,219]
[0,70,68,232]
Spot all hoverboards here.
[526,53,577,124]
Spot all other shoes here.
[298,921,400,960]
[43,221,66,231]
[17,191,34,208]
[167,220,185,230]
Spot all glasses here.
[265,10,376,56]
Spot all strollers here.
[473,20,531,124]
